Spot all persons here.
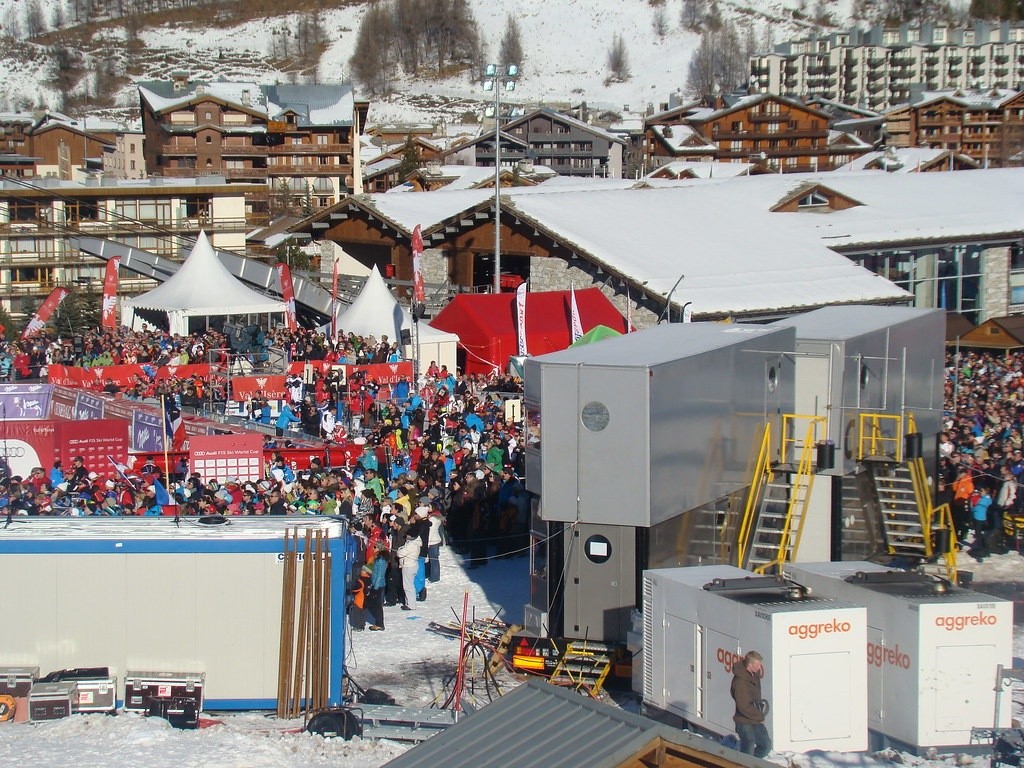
[0,321,535,631]
[729,650,772,758]
[935,350,1024,558]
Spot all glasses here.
[269,494,278,498]
[243,495,249,497]
[388,519,393,522]
[198,500,203,502]
[373,546,378,549]
[309,492,318,495]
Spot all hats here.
[57,482,69,491]
[419,496,430,505]
[211,482,221,491]
[314,473,322,481]
[502,467,513,475]
[146,485,156,493]
[275,456,284,462]
[486,463,494,471]
[361,562,374,574]
[258,481,270,490]
[354,522,363,531]
[394,517,405,526]
[414,506,429,518]
[360,489,373,498]
[463,443,472,450]
[88,472,98,479]
[399,487,409,495]
[341,477,350,484]
[429,488,438,496]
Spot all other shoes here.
[369,625,385,630]
[401,604,411,610]
[419,587,427,601]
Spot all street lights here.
[482,65,520,294]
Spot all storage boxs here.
[27,683,81,723]
[62,676,118,712]
[168,697,198,728]
[0,667,40,698]
[145,696,171,717]
[123,672,206,711]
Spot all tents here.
[121,228,289,338]
[309,262,459,391]
[429,287,636,380]
